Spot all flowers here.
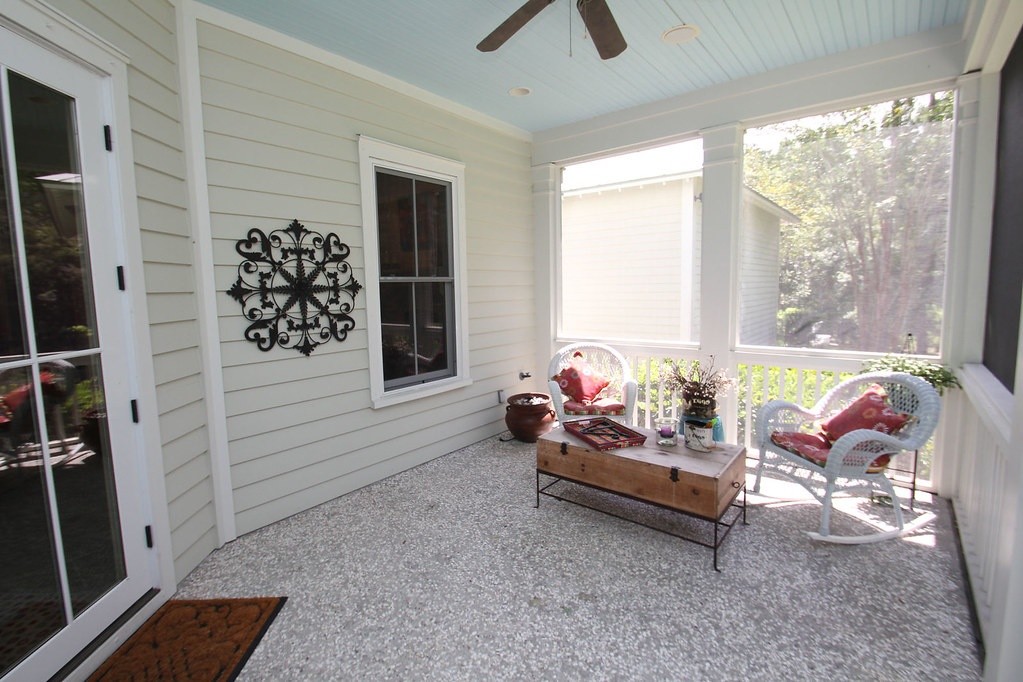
[659,354,737,419]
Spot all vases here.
[505,392,556,443]
[679,411,725,442]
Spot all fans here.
[476,0,627,61]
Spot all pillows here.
[821,384,911,440]
[551,351,611,408]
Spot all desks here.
[534,416,751,573]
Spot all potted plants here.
[859,358,963,422]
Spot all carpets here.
[84,597,287,682]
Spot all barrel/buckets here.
[683,419,717,453]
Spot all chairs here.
[753,370,939,545]
[545,341,638,425]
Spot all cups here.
[654,417,680,447]
